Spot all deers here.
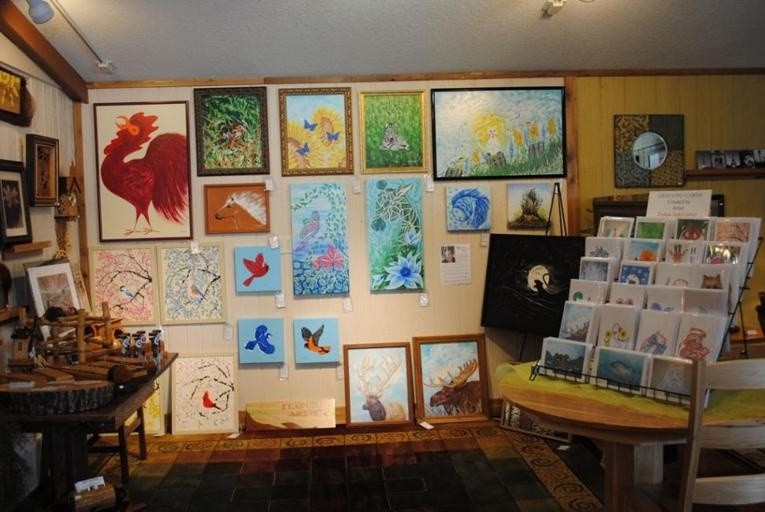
[423,357,481,417]
[355,355,406,421]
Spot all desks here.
[0,351,179,482]
[499,358,764,512]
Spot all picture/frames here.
[192,86,271,178]
[411,330,490,425]
[23,255,82,352]
[92,100,195,243]
[278,86,355,177]
[357,89,431,176]
[155,241,229,326]
[0,65,61,249]
[430,86,570,182]
[202,182,271,236]
[86,243,162,327]
[97,373,166,438]
[342,341,416,431]
[170,351,239,437]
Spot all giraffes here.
[369,179,423,290]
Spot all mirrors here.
[613,113,687,189]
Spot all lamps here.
[26,0,55,25]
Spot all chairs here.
[620,355,765,512]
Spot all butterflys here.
[296,143,309,156]
[304,119,318,131]
[325,131,340,142]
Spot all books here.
[578,257,741,329]
[597,218,760,278]
[569,278,730,351]
[540,337,709,407]
[558,301,730,367]
[585,237,751,302]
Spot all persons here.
[441,246,456,263]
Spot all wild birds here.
[300,324,331,356]
[118,285,137,301]
[203,391,222,411]
[244,324,275,354]
[190,284,207,300]
[241,252,270,287]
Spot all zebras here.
[378,120,410,153]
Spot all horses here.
[213,190,268,233]
